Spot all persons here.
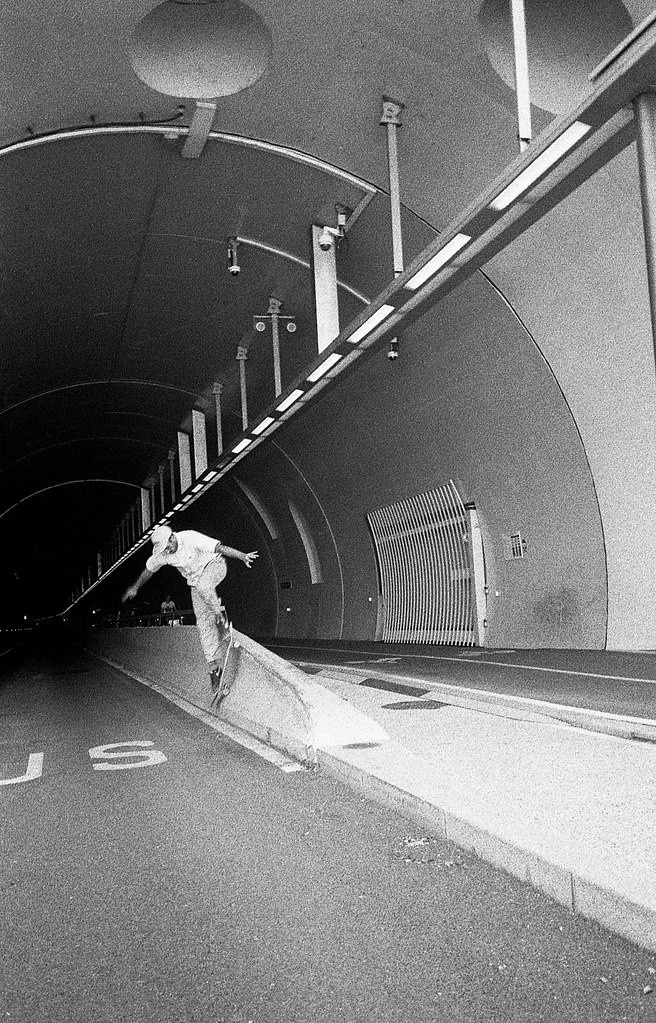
[160,593,178,613]
[121,526,260,693]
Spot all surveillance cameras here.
[230,265,240,276]
[319,235,333,251]
[388,350,399,361]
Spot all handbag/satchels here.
[169,617,184,626]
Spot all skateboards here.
[210,620,240,709]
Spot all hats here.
[151,526,172,559]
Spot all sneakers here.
[209,665,222,693]
[216,613,231,642]
